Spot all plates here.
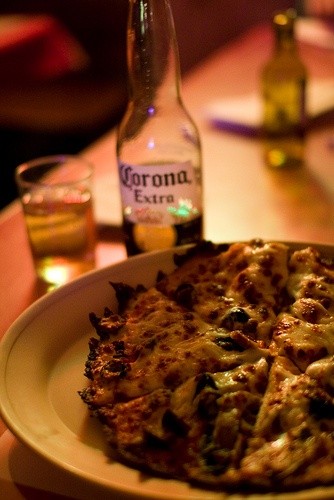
[0,241,332,500]
[211,78,334,127]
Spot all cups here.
[16,157,98,292]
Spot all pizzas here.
[78,236,334,495]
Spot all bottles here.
[116,1,203,258]
[261,9,307,171]
